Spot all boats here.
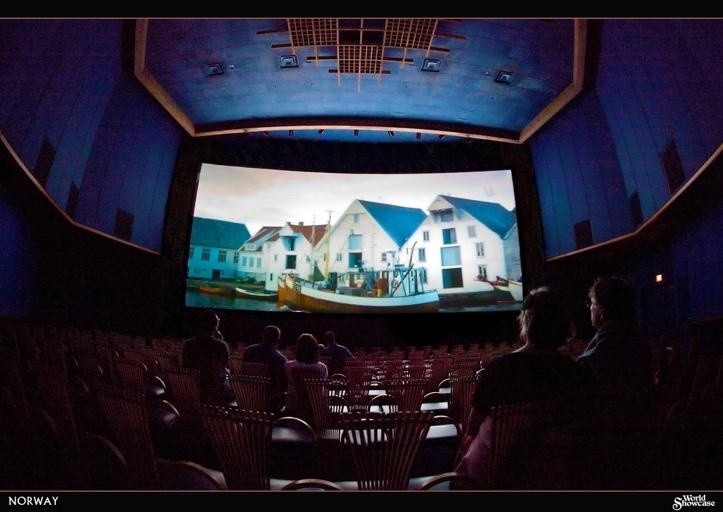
[195,283,232,296]
[475,272,523,303]
[495,274,522,302]
[275,265,442,313]
[233,287,279,302]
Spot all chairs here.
[0,316,723,490]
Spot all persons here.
[455,283,584,486]
[577,275,655,412]
[284,331,326,414]
[183,311,236,404]
[242,325,288,414]
[319,331,356,373]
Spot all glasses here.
[586,301,598,308]
